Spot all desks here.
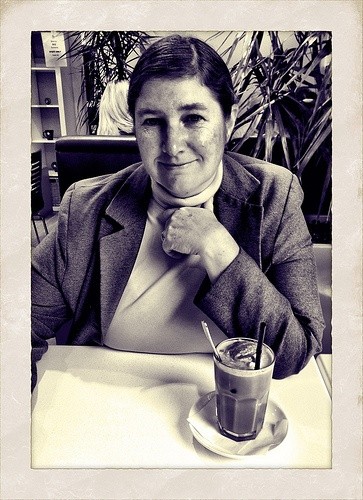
[32,343,332,468]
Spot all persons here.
[96,79,134,136]
[31,34,326,394]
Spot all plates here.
[188,390,290,460]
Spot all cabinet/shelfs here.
[31,65,76,210]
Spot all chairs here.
[31,149,49,245]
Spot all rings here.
[162,229,165,238]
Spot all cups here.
[212,336,276,443]
[43,130,54,140]
